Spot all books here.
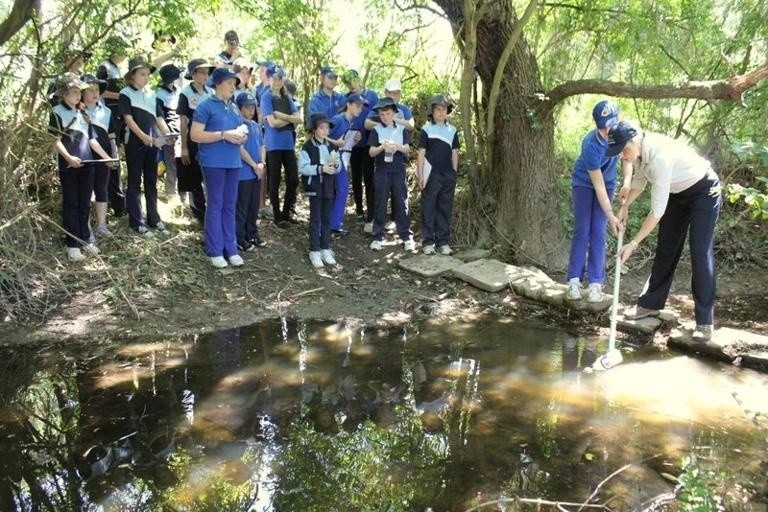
[67,156,121,170]
[270,97,294,131]
[415,158,433,189]
[106,77,125,108]
[338,128,363,152]
[139,132,183,151]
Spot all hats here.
[384,78,402,92]
[151,29,175,48]
[305,113,335,131]
[101,34,133,58]
[265,65,287,78]
[232,58,255,74]
[425,94,453,116]
[48,71,89,103]
[591,100,619,130]
[605,119,638,157]
[53,42,92,65]
[346,93,369,106]
[223,30,240,41]
[237,92,258,106]
[157,64,184,87]
[342,69,359,83]
[319,66,338,79]
[284,79,298,102]
[204,67,241,88]
[182,58,216,79]
[256,58,274,69]
[371,97,398,113]
[81,74,107,96]
[124,56,156,80]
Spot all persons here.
[49,71,120,262]
[236,93,267,252]
[255,60,273,122]
[97,34,134,81]
[567,101,632,303]
[156,64,188,202]
[46,42,96,96]
[366,97,415,252]
[149,30,184,89]
[190,67,249,269]
[364,78,415,231]
[328,94,369,236]
[177,58,216,216]
[260,64,308,231]
[216,29,243,65]
[303,379,341,430]
[285,79,303,143]
[298,113,343,269]
[80,74,121,245]
[418,95,460,256]
[234,57,256,91]
[118,58,181,239]
[341,69,379,233]
[308,67,346,118]
[605,120,721,341]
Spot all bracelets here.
[260,161,267,168]
[630,240,639,249]
[220,130,226,141]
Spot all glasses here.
[159,38,173,44]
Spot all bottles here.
[330,149,336,168]
[333,151,340,168]
[384,136,393,162]
[584,350,622,374]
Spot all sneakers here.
[236,240,252,252]
[272,218,287,228]
[308,251,324,269]
[247,235,267,248]
[622,304,660,320]
[129,222,148,234]
[363,221,375,234]
[209,256,226,269]
[97,226,113,237]
[358,212,368,217]
[65,245,81,261]
[328,230,345,237]
[420,246,436,257]
[436,244,454,255]
[370,239,382,251]
[80,243,103,257]
[287,212,307,226]
[149,221,171,236]
[384,221,398,231]
[403,238,414,254]
[258,207,271,221]
[588,282,604,304]
[227,254,243,267]
[693,324,714,341]
[566,278,583,301]
[321,248,337,266]
